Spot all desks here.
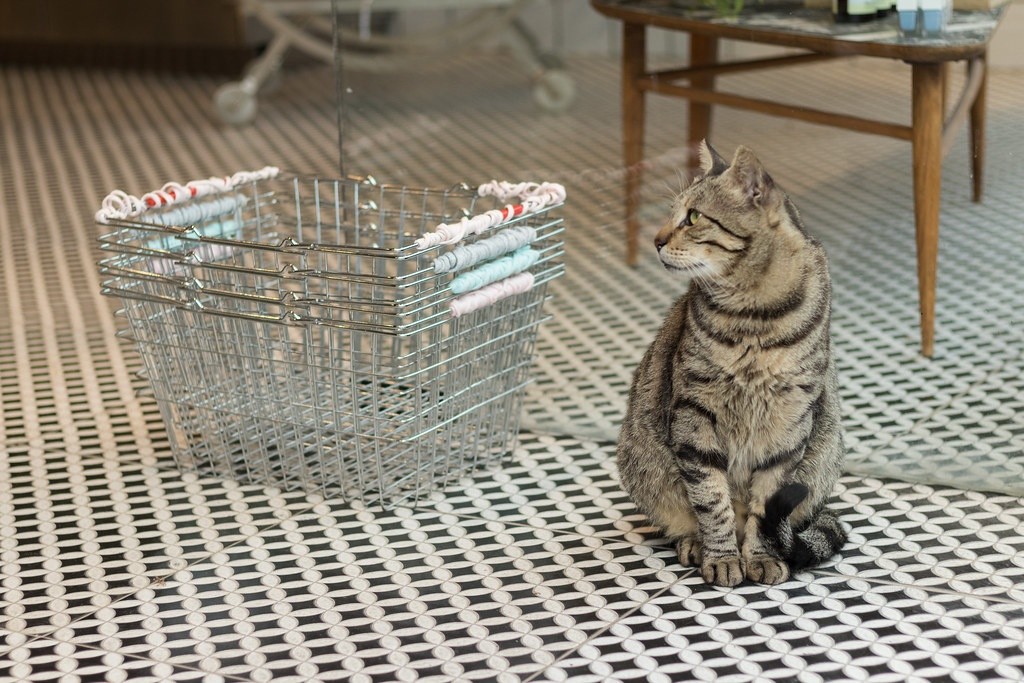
[588,0,1013,356]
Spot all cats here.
[615,137,849,586]
